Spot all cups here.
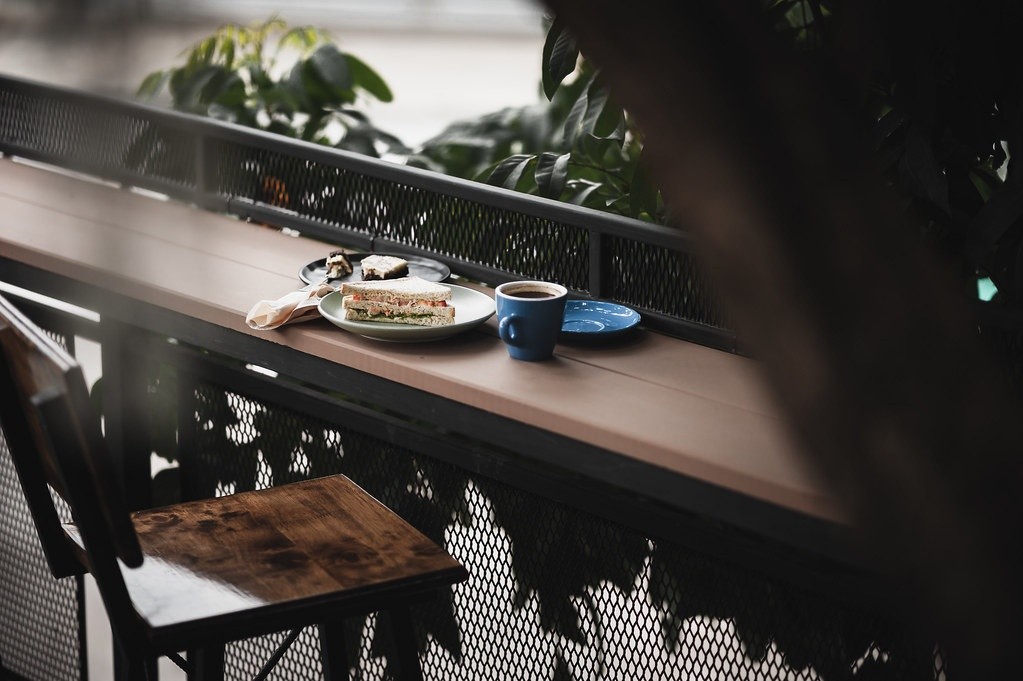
[494,280,569,362]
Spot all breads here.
[359,254,409,280]
[326,251,353,281]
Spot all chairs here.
[0,292,468,681]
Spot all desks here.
[0,155,871,559]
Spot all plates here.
[298,251,451,286]
[317,282,496,343]
[561,299,641,339]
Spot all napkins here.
[244,282,334,331]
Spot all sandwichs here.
[340,276,457,327]
